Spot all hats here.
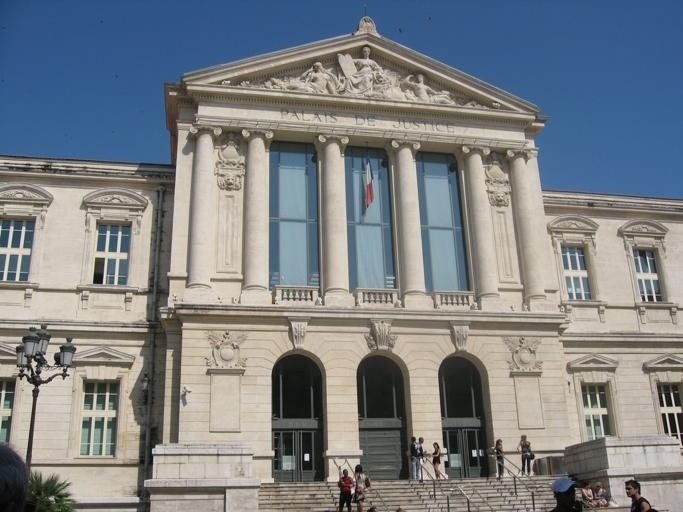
[551,476,578,494]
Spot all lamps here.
[139,370,149,405]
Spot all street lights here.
[14,324,76,500]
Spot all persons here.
[592,482,607,507]
[270,60,336,96]
[415,436,430,480]
[549,477,583,511]
[492,438,504,477]
[361,16,373,32]
[410,435,416,480]
[352,464,370,511]
[516,434,534,476]
[401,72,459,107]
[578,482,597,507]
[623,479,652,512]
[337,467,354,511]
[340,46,383,97]
[431,441,441,480]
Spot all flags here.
[363,159,374,207]
